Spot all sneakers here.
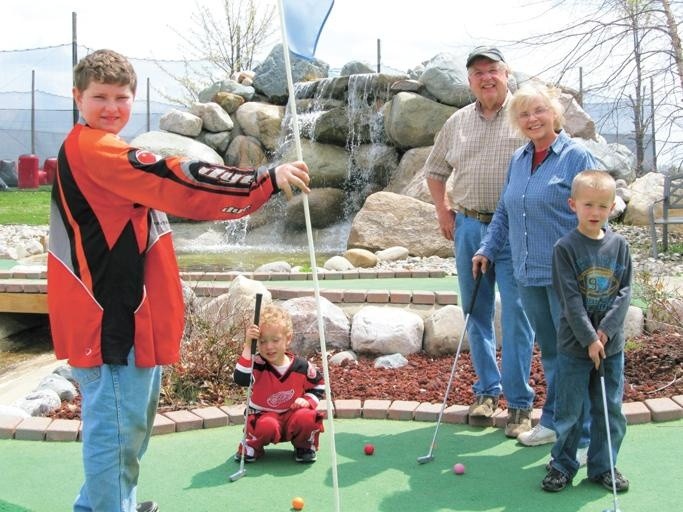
[539,466,574,493]
[233,444,265,462]
[504,406,532,438]
[467,396,499,418]
[294,446,316,463]
[517,423,557,447]
[136,500,161,512]
[546,445,591,471]
[589,466,630,494]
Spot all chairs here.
[647,174,683,258]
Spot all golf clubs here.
[417,263,480,466]
[229,293,262,481]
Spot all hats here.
[464,45,505,67]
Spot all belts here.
[458,205,494,223]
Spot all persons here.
[540,170,633,492]
[46,45,311,512]
[233,304,325,464]
[473,84,598,470]
[422,44,537,438]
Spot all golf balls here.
[453,462,464,474]
[293,496,304,510]
[364,443,374,455]
